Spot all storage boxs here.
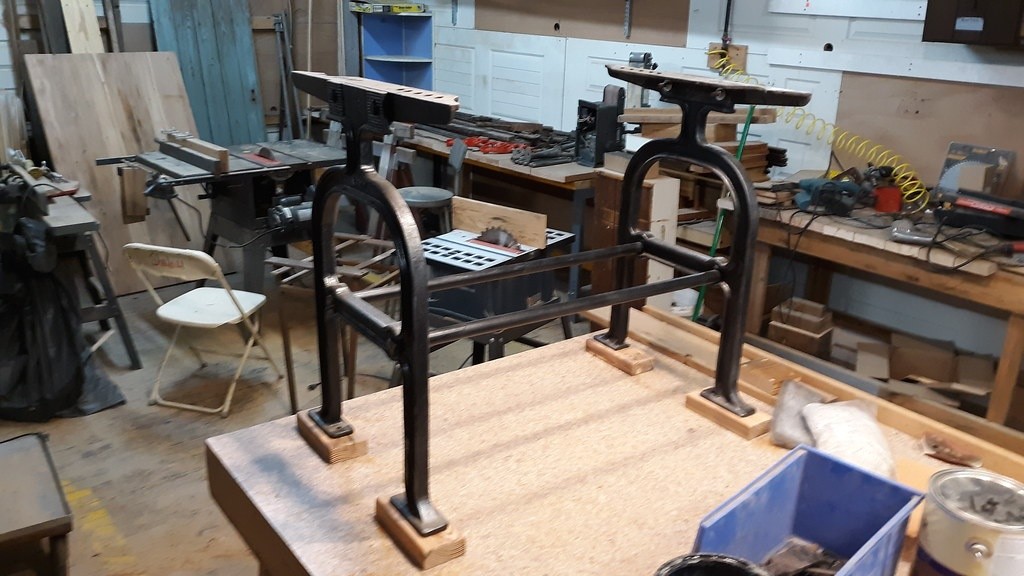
[348,0,425,14]
[689,444,927,576]
[855,332,995,409]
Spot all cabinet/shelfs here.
[361,10,435,93]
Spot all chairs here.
[122,242,286,419]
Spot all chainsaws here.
[421,228,576,344]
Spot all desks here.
[208,326,960,576]
[716,170,1024,427]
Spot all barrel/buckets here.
[906,468,1024,576]
[265,125,280,141]
